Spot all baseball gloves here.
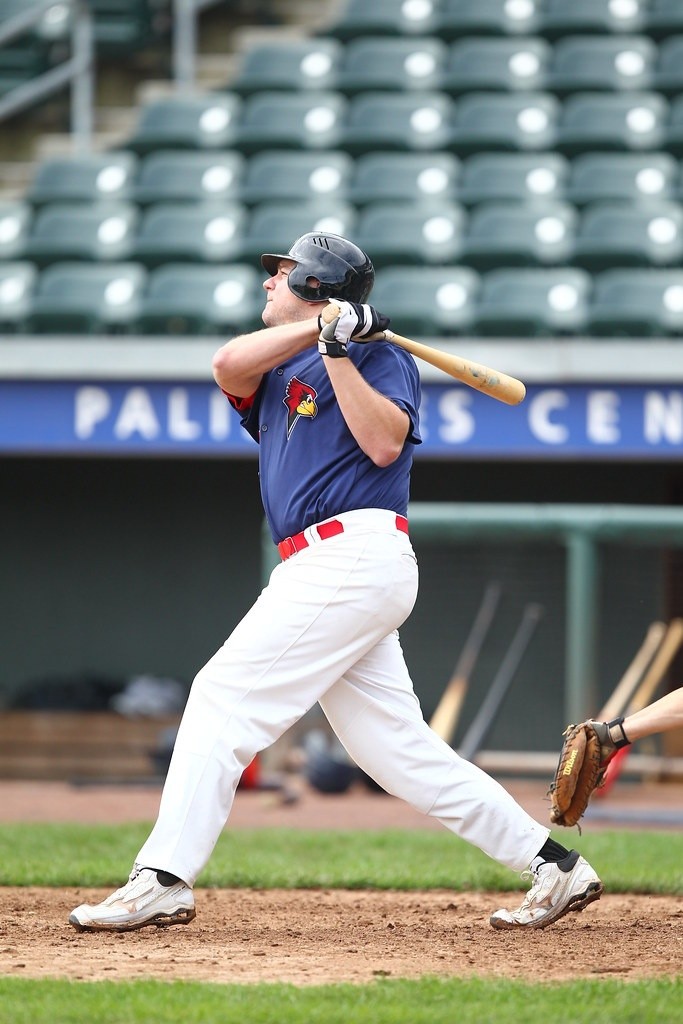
[542,717,632,836]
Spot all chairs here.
[0,0,683,337]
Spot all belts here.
[278,515,409,562]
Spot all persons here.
[547,685,683,826]
[67,231,604,932]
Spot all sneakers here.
[489,849,604,929]
[70,869,196,932]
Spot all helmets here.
[261,232,375,304]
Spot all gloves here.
[317,297,390,358]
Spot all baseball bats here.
[322,304,527,406]
